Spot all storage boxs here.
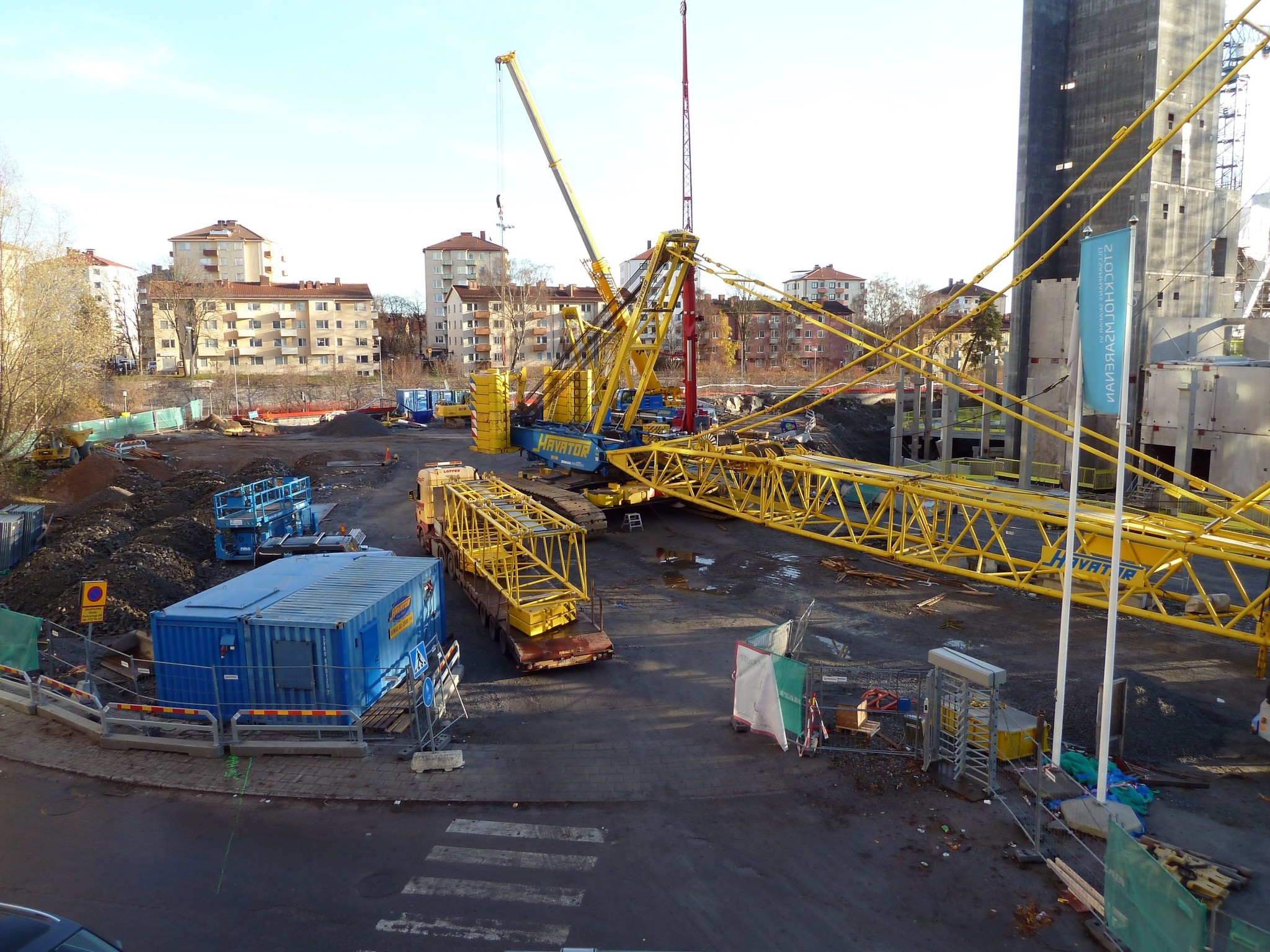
[899,697,911,712]
[835,695,867,729]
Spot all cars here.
[508,390,542,405]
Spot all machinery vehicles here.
[429,49,747,543]
[432,366,528,429]
[98,353,137,378]
[26,424,97,470]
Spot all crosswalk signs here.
[409,640,429,680]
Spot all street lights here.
[231,344,239,416]
[207,379,214,414]
[119,389,132,437]
[219,369,225,417]
[975,351,985,394]
[245,362,252,412]
[376,336,383,408]
[185,326,196,401]
[390,358,394,407]
[811,348,817,389]
[839,361,843,383]
[726,336,759,392]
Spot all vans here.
[147,361,156,375]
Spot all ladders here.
[621,513,644,532]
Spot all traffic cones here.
[385,446,390,462]
[340,524,346,535]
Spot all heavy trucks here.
[408,460,617,676]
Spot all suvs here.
[252,532,396,569]
